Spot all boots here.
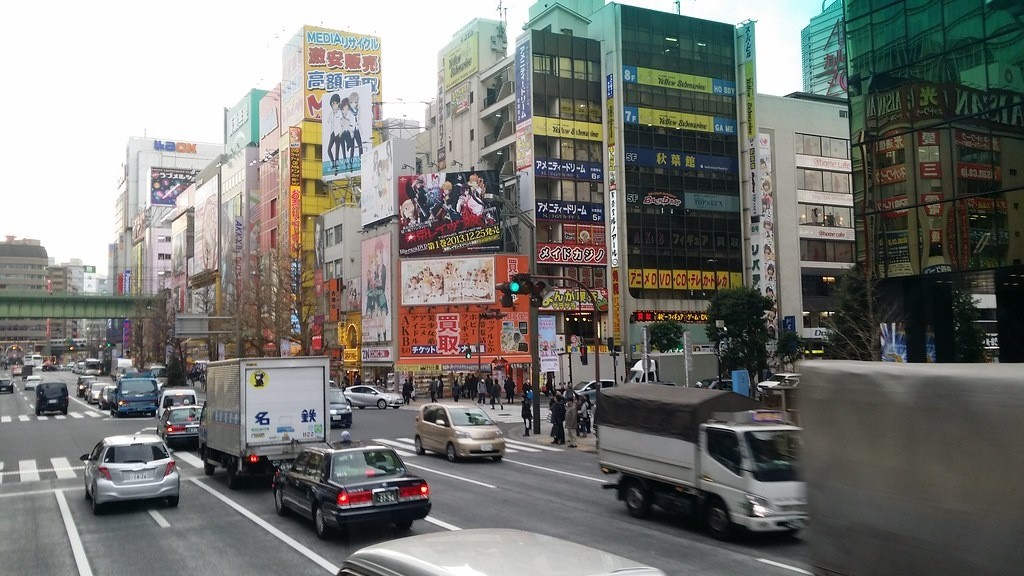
[560,437,566,445]
[523,427,529,437]
[550,437,558,444]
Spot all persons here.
[343,371,387,392]
[426,370,516,410]
[543,373,595,447]
[402,379,416,405]
[187,362,205,385]
[521,377,534,436]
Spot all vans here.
[155,386,197,422]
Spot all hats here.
[481,378,484,382]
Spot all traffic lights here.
[579,344,587,365]
[495,281,514,308]
[465,348,471,359]
[106,343,116,347]
[69,346,75,350]
[508,280,534,294]
[530,280,547,307]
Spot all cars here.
[12,352,170,417]
[563,377,619,403]
[23,374,41,391]
[329,387,353,428]
[79,433,181,514]
[343,384,406,409]
[754,373,801,399]
[270,441,433,541]
[35,381,69,417]
[155,402,205,448]
[0,377,14,393]
[336,526,671,576]
[415,402,507,461]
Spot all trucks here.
[190,354,333,491]
[594,379,810,542]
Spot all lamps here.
[451,160,463,167]
[496,113,501,116]
[402,164,414,169]
[497,151,503,154]
[428,162,437,167]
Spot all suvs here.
[697,378,733,391]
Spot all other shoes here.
[483,403,485,406]
[492,407,495,410]
[501,406,504,409]
[476,403,479,405]
